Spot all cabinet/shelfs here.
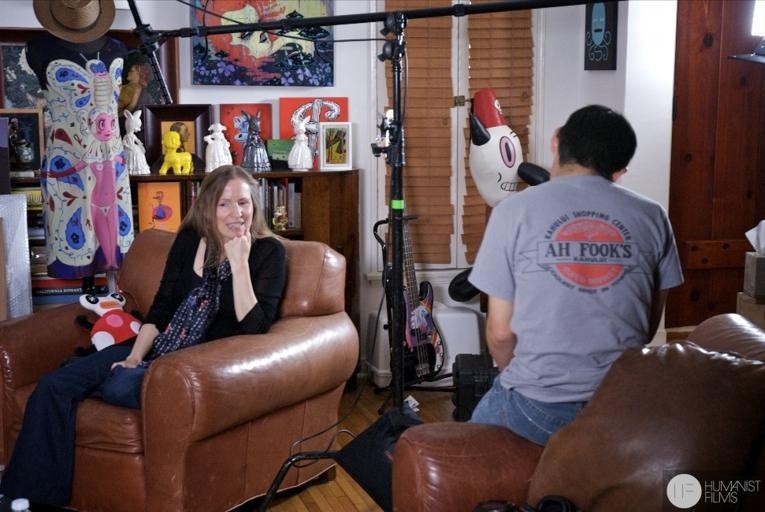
[129,175,359,255]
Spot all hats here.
[32,0,117,45]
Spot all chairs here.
[388,305,764,510]
[0,228,362,512]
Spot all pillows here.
[517,330,764,512]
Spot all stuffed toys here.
[79,292,143,352]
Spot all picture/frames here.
[319,122,352,172]
[145,102,212,174]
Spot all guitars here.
[375,106,444,385]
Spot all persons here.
[161,122,191,151]
[467,105,685,446]
[0,165,286,512]
[118,64,151,117]
[49,108,128,270]
